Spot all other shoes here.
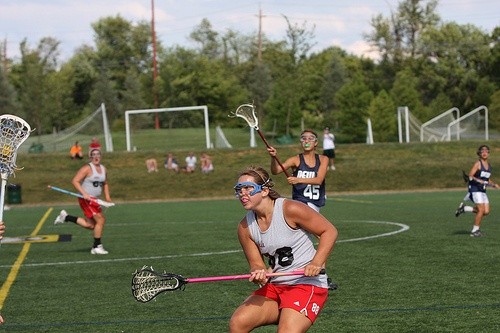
[328,282,337,290]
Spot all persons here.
[69,140,84,160]
[143,155,159,172]
[89,138,101,157]
[322,126,337,171]
[53,149,113,255]
[229,167,322,317]
[198,152,215,173]
[455,144,499,238]
[183,153,197,172]
[266,129,330,241]
[0,219,6,324]
[164,154,180,172]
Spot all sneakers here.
[456,202,465,217]
[91,244,108,254]
[470,230,482,237]
[54,210,68,224]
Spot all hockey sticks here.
[462,170,495,186]
[0,114,31,222]
[131,268,326,303]
[235,104,289,177]
[47,185,116,208]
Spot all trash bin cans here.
[7,184,21,203]
